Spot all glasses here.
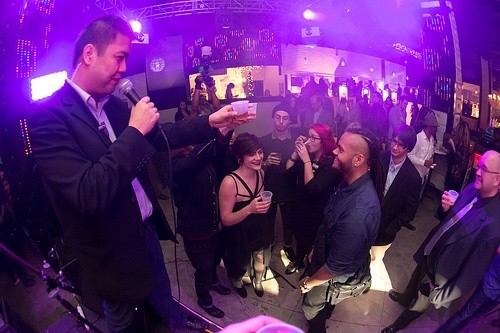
[476,165,500,174]
[390,138,406,146]
[305,134,321,141]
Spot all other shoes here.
[402,223,416,230]
[282,246,296,262]
[254,279,264,297]
[389,289,410,308]
[234,282,247,298]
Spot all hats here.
[272,104,297,123]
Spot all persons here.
[462,98,473,117]
[164,73,437,333]
[296,127,381,333]
[382,149,500,333]
[25,15,256,333]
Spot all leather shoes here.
[285,262,305,275]
[214,286,231,295]
[197,300,225,319]
[184,316,209,330]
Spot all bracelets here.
[289,156,296,163]
[303,277,312,291]
[303,161,311,164]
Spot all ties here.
[424,196,479,255]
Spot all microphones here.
[117,78,161,128]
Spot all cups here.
[304,140,312,150]
[260,190,273,203]
[231,100,250,122]
[449,190,459,206]
[271,153,281,168]
